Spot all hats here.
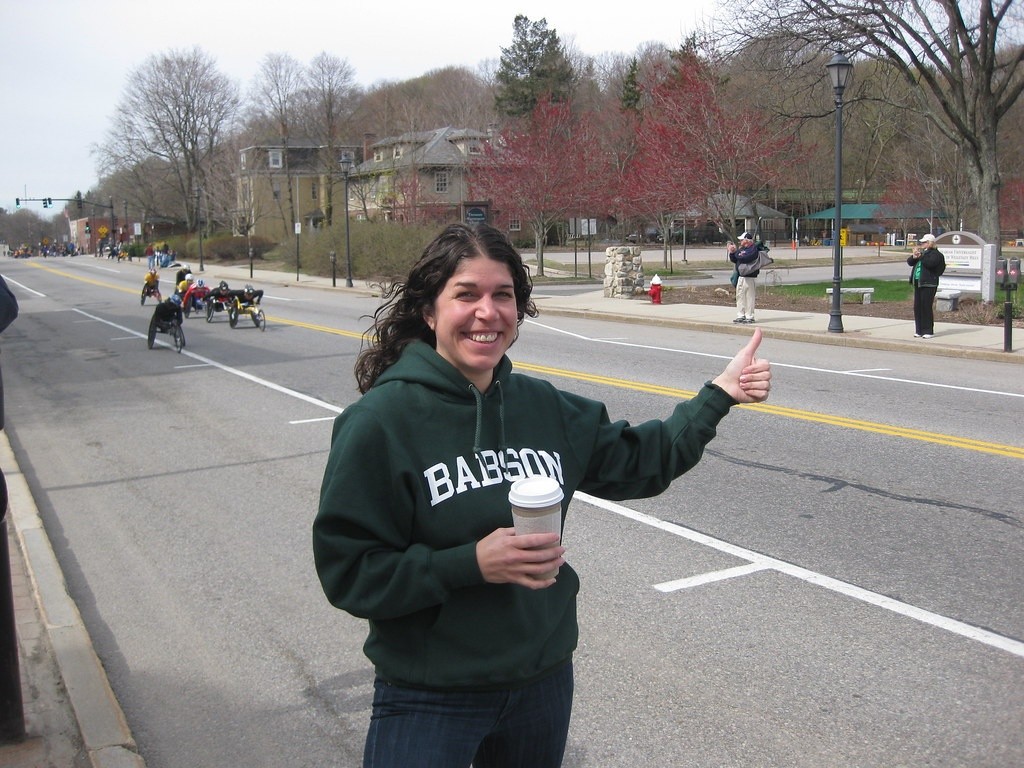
[919,233,936,242]
[737,231,752,241]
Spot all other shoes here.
[743,318,755,324]
[733,316,746,323]
[923,333,934,339]
[914,333,921,337]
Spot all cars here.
[627,221,729,246]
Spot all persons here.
[312,221,772,768]
[145,241,176,270]
[182,279,211,309]
[178,274,195,293]
[907,234,946,339]
[175,264,191,288]
[144,268,159,296]
[43,241,74,258]
[727,232,760,324]
[98,238,123,262]
[203,281,235,308]
[228,285,263,305]
[155,295,183,331]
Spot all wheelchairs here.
[140,281,162,305]
[174,285,234,323]
[147,310,186,354]
[228,296,266,332]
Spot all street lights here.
[192,183,205,272]
[338,152,354,287]
[122,197,130,254]
[924,178,944,235]
[824,44,855,333]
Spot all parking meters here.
[248,248,254,278]
[329,251,337,287]
[155,246,159,266]
[995,255,1021,353]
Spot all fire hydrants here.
[646,273,664,306]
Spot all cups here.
[508,474,565,580]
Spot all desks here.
[896,240,904,245]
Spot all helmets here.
[182,264,254,296]
[149,268,156,276]
[170,295,182,305]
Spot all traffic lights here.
[114,217,119,226]
[42,198,48,208]
[15,197,20,208]
[77,198,82,208]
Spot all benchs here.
[826,287,874,305]
[935,289,962,311]
[766,241,771,247]
